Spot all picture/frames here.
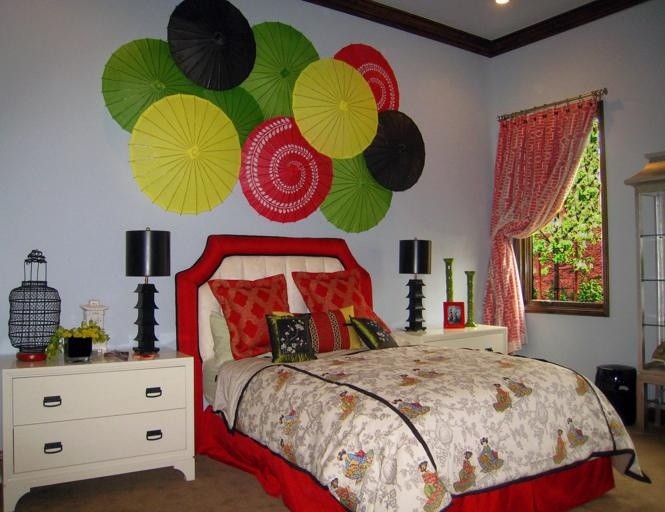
[443,300,467,329]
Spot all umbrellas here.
[129,94,241,216]
[320,154,395,233]
[237,118,334,223]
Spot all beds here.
[169,231,653,512]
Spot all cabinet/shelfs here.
[621,147,665,437]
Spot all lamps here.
[399,233,434,335]
[123,224,172,356]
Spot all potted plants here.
[46,319,111,361]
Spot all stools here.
[592,362,639,428]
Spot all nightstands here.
[0,342,201,512]
[394,322,511,355]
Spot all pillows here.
[256,272,309,312]
[263,313,319,364]
[348,315,401,350]
[290,267,391,336]
[208,309,235,361]
[207,272,292,360]
[306,305,361,351]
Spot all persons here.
[448,308,454,321]
[454,311,459,322]
